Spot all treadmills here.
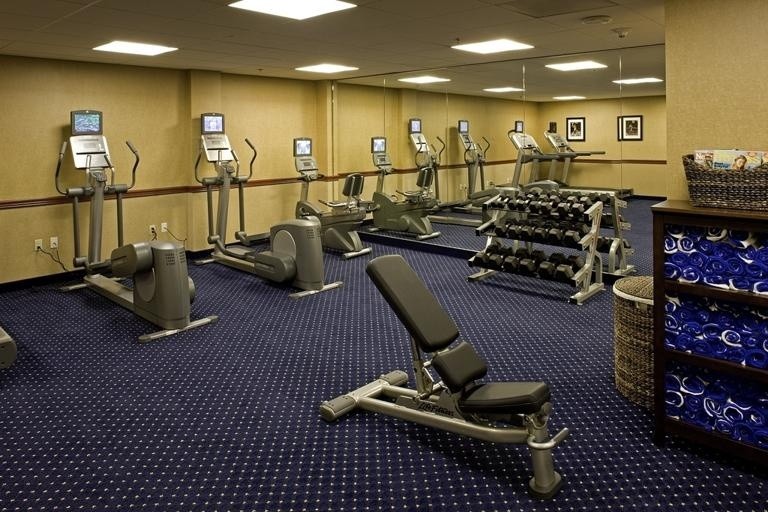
[509,132,635,198]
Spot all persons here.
[209,120,216,129]
[731,155,747,170]
[572,123,577,135]
[627,123,633,133]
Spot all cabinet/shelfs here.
[652,196,768,456]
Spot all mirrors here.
[329,44,666,279]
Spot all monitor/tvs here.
[410,119,421,133]
[459,120,468,133]
[550,123,556,133]
[515,121,523,133]
[294,138,312,156]
[202,113,224,134]
[372,137,386,153]
[72,110,102,135]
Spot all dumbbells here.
[597,213,623,252]
[475,243,581,280]
[495,218,587,245]
[490,191,612,214]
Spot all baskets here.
[682,154,768,211]
